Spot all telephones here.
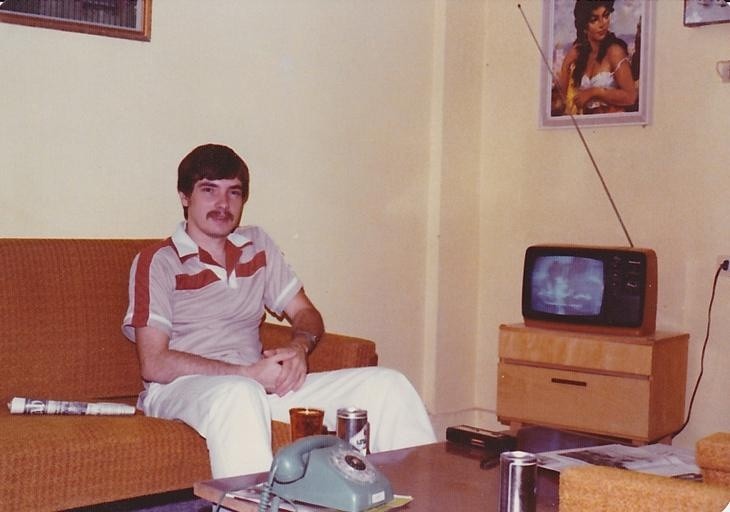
[258,435,394,512]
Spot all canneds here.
[499,451,538,511]
[336,408,368,458]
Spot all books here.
[637,442,700,469]
[531,439,699,486]
[224,479,414,511]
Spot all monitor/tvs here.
[521,245,658,336]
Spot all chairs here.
[558,433,730,512]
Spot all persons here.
[534,259,563,313]
[562,254,598,316]
[554,0,640,118]
[119,139,442,512]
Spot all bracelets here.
[289,342,311,358]
[294,329,319,346]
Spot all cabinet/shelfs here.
[497,324,690,447]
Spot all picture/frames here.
[0,0,153,43]
[537,1,654,129]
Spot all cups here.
[289,408,324,445]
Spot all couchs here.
[1,240,380,512]
